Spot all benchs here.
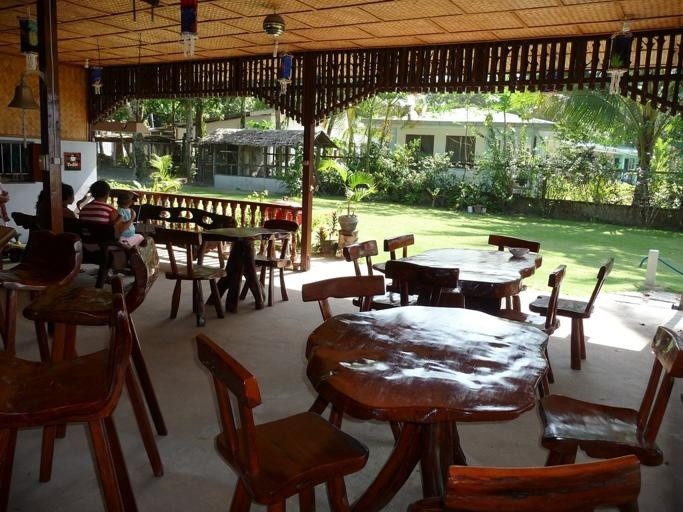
[13,211,147,288]
[111,204,237,266]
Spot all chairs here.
[240,220,299,307]
[302,276,403,442]
[195,333,368,512]
[407,452,642,512]
[155,227,227,325]
[541,327,683,466]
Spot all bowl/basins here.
[508,248,530,258]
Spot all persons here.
[0,179,10,227]
[74,191,95,218]
[61,184,76,217]
[79,180,142,251]
[117,190,138,239]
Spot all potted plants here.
[317,160,380,231]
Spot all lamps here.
[18,6,40,74]
[605,13,632,97]
[279,50,294,95]
[181,0,198,58]
[92,64,103,95]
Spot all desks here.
[201,229,289,313]
[306,306,550,511]
